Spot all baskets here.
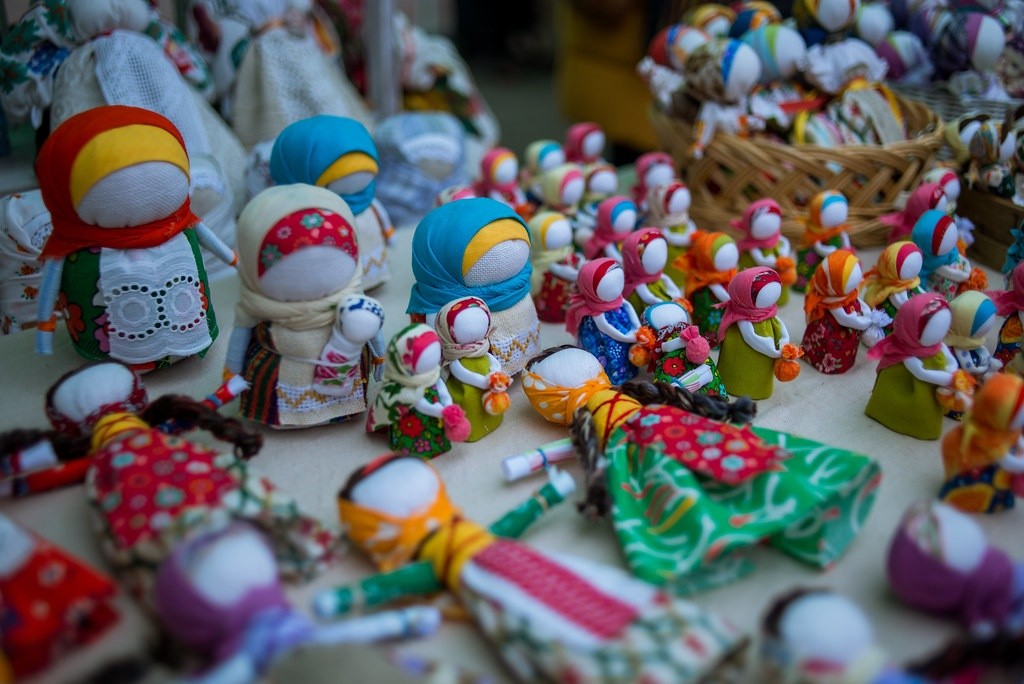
[899,87,1014,165]
[656,80,945,249]
